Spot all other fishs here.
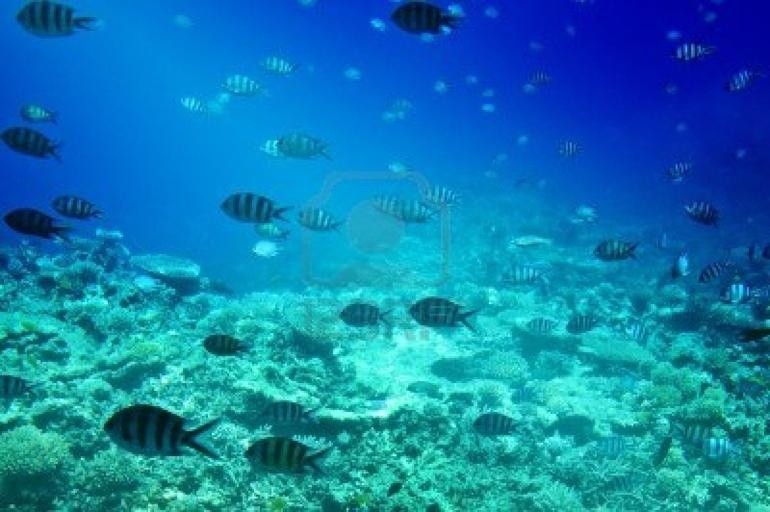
[390,1,466,35]
[171,40,769,341]
